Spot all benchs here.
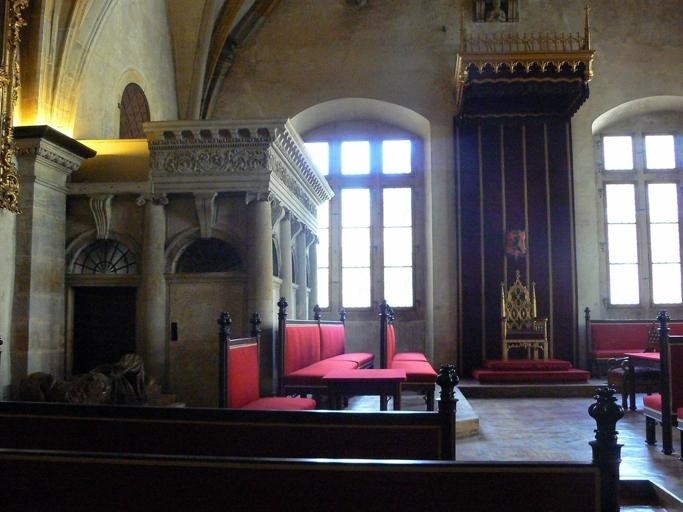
[642,311,683,455]
[587,306,683,379]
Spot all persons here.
[486,0,506,22]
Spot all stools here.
[320,368,408,410]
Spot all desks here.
[622,351,660,411]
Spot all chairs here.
[607,320,660,410]
[499,268,548,361]
[379,300,439,412]
[218,310,317,412]
[277,297,376,410]
